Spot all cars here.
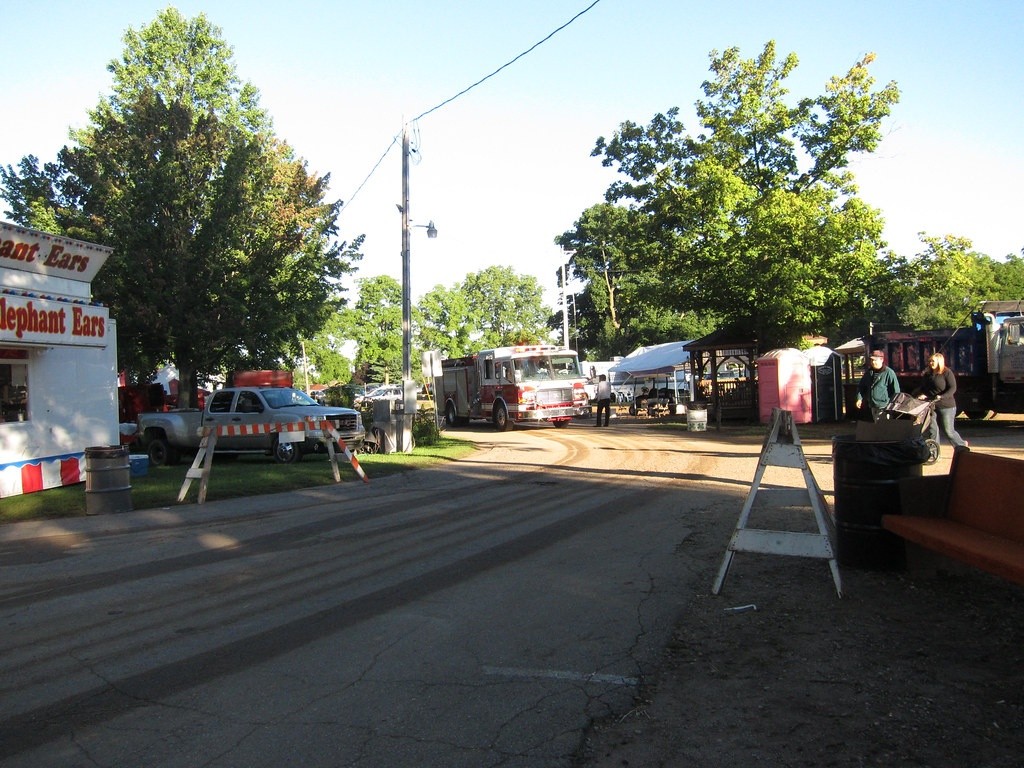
[293,382,433,411]
[578,356,759,415]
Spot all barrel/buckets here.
[686,402,708,433]
[829,434,924,568]
[85,445,131,517]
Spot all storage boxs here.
[128,455,150,479]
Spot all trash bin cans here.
[85,445,133,516]
[830,433,930,574]
[686,401,709,432]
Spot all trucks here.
[874,299,1024,421]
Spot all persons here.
[914,352,968,463]
[592,374,611,427]
[855,350,900,424]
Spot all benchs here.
[881,449,1024,589]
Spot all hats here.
[870,350,884,359]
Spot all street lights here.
[402,219,437,381]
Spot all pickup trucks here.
[136,386,366,464]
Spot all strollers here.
[877,393,941,465]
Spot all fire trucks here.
[434,345,593,429]
